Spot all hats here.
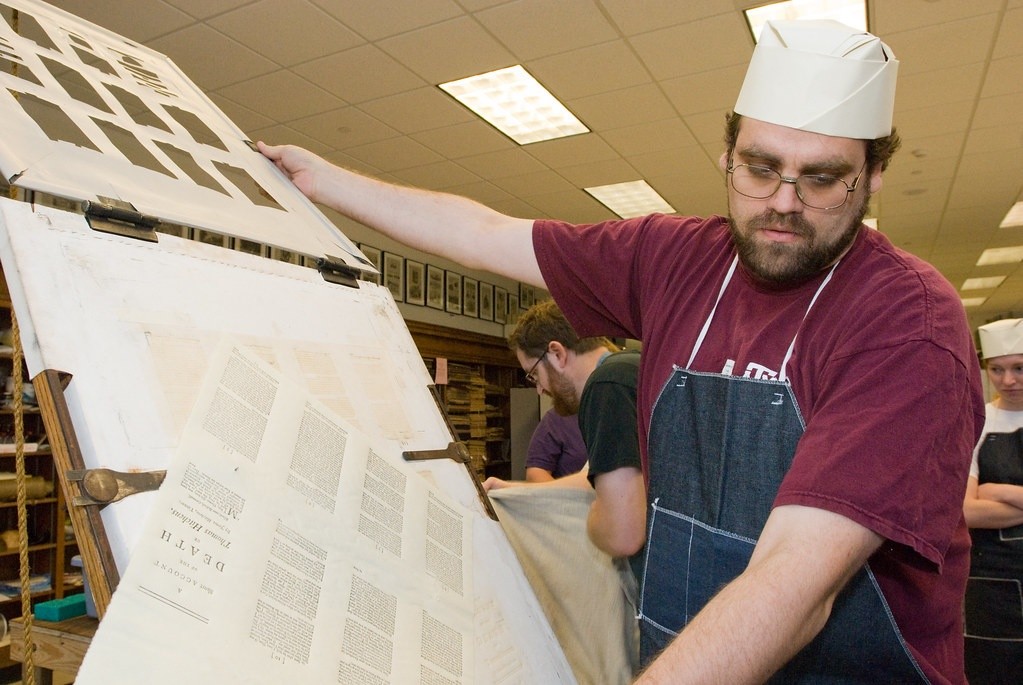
[732,17,900,140]
[977,318,1023,360]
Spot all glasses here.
[724,148,869,210]
[525,348,550,384]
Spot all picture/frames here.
[507,293,519,316]
[478,280,494,322]
[444,270,463,316]
[425,263,445,312]
[404,258,426,307]
[493,285,508,325]
[526,285,535,311]
[29,190,383,287]
[518,282,528,310]
[381,250,406,304]
[462,274,479,319]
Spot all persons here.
[525,407,588,483]
[257,18,987,685]
[481,297,647,557]
[962,318,1023,685]
